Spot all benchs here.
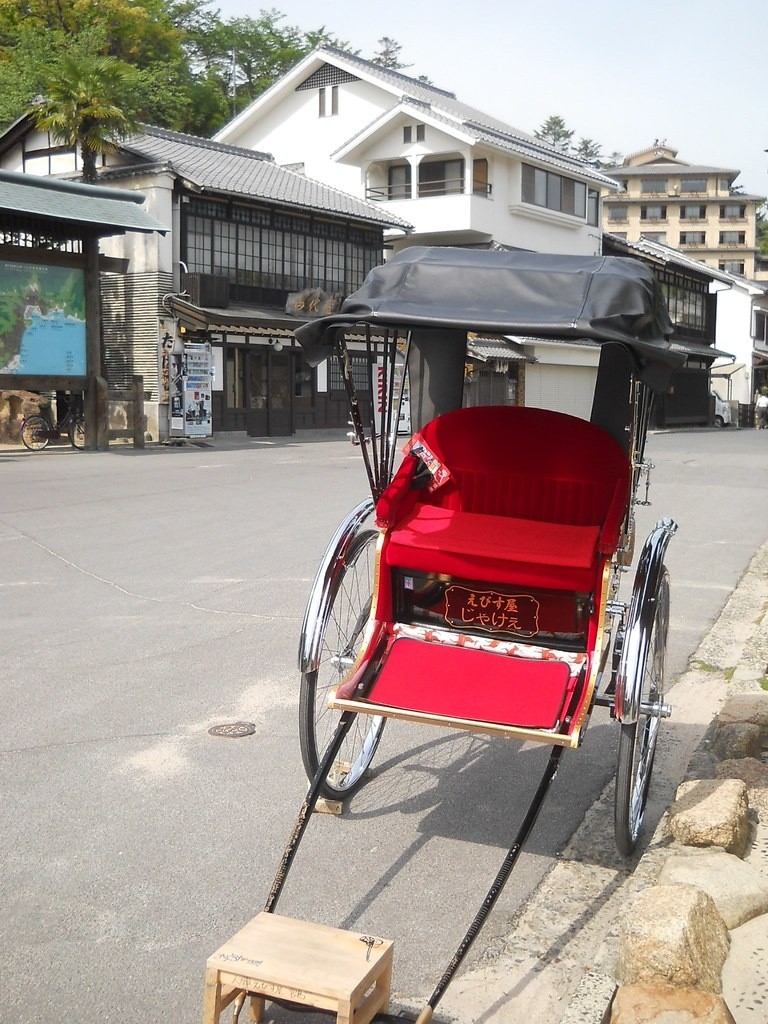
[375,406,631,593]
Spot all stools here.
[204,911,396,1024]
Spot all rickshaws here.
[262,244,696,1024]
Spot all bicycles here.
[20,394,89,451]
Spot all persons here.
[755,392,768,430]
[754,388,762,430]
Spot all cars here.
[711,390,731,428]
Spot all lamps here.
[274,340,283,351]
